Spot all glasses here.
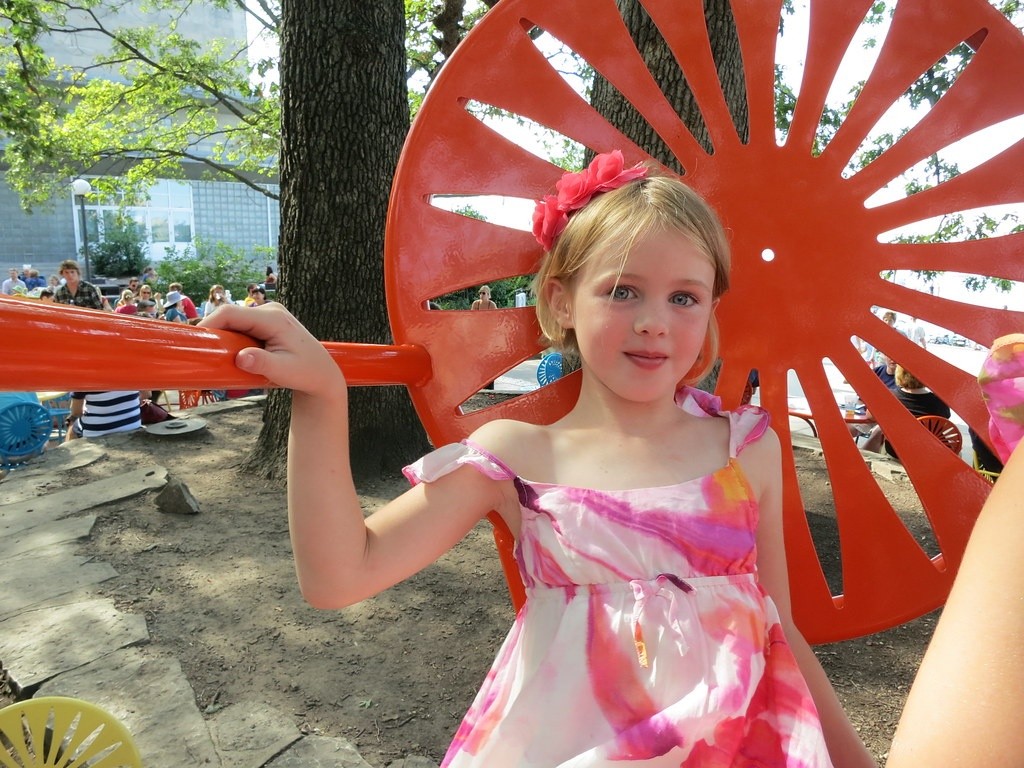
[143,291,151,294]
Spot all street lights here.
[71,179,91,282]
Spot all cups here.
[844,395,857,415]
[215,293,221,300]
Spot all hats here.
[164,292,188,309]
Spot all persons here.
[854,335,874,361]
[64,389,153,444]
[845,355,902,438]
[0,261,278,330]
[871,312,908,366]
[882,330,1022,768]
[902,315,927,349]
[188,145,879,768]
[863,364,953,462]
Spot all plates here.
[165,422,187,428]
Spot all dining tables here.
[785,394,877,451]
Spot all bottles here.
[69,300,75,305]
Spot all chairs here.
[918,414,963,458]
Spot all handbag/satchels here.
[138,399,167,424]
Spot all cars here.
[926,324,979,349]
